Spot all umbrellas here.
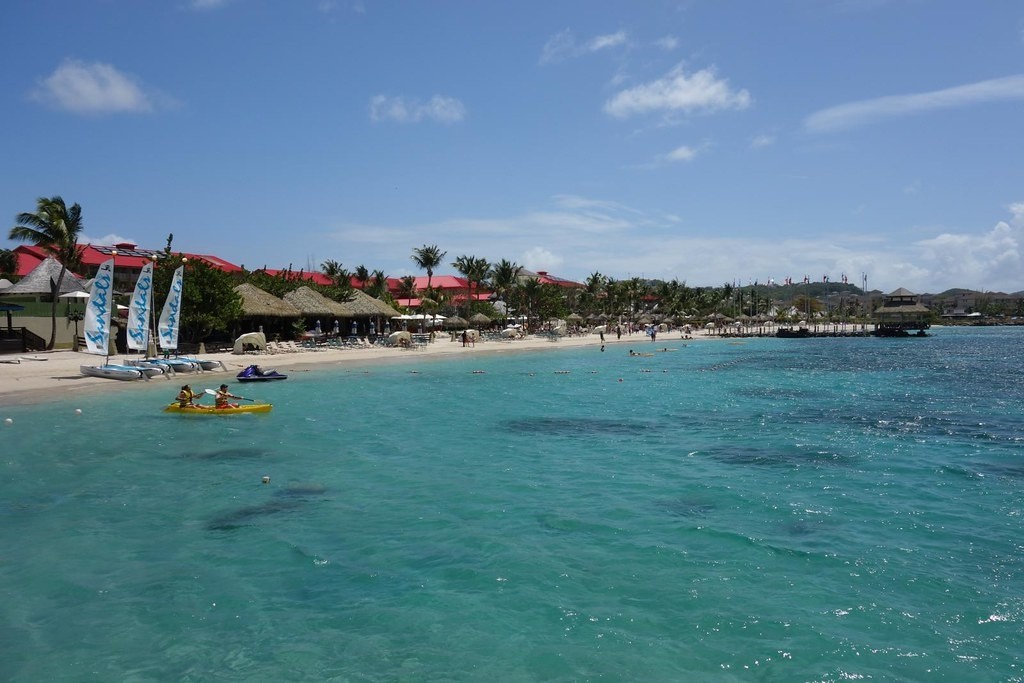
[311,313,490,335]
[567,312,771,328]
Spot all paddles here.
[155,363,172,380]
[164,361,176,377]
[218,360,228,372]
[187,356,198,374]
[204,388,266,405]
[170,386,220,405]
[133,363,150,382]
[195,356,204,373]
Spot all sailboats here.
[79,250,221,381]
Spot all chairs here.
[267,333,428,355]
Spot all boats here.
[163,402,272,414]
[235,365,289,383]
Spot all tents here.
[680,321,807,329]
[502,328,517,340]
[458,329,480,343]
[225,282,401,334]
[592,324,607,333]
[233,333,268,355]
[388,331,414,349]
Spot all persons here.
[162,348,170,360]
[523,322,741,355]
[460,331,475,348]
[174,384,205,408]
[214,384,244,409]
